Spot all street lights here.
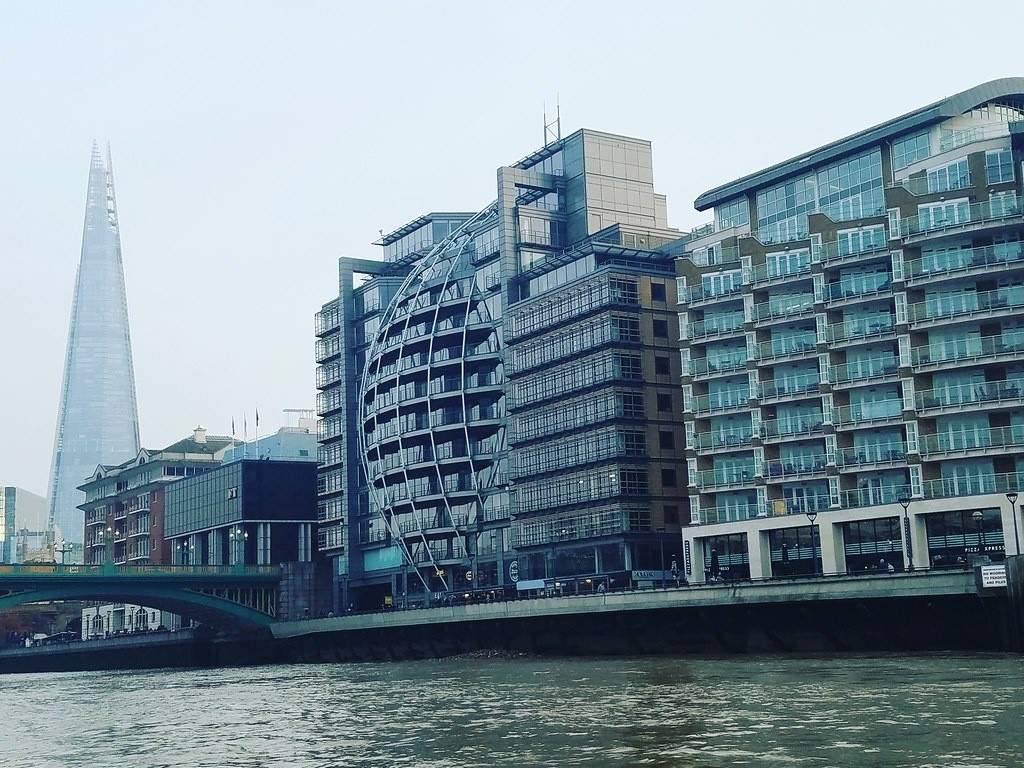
[468,552,475,600]
[65,617,69,644]
[130,607,135,631]
[898,497,916,572]
[805,511,819,576]
[342,573,350,609]
[657,527,668,588]
[86,614,91,639]
[176,540,195,565]
[1005,493,1021,556]
[230,529,248,567]
[107,610,111,635]
[54,538,73,563]
[399,564,407,608]
[99,526,120,566]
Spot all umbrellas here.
[671,554,681,587]
[709,548,720,583]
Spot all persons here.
[934,554,946,565]
[706,571,725,585]
[597,580,605,593]
[877,558,894,574]
